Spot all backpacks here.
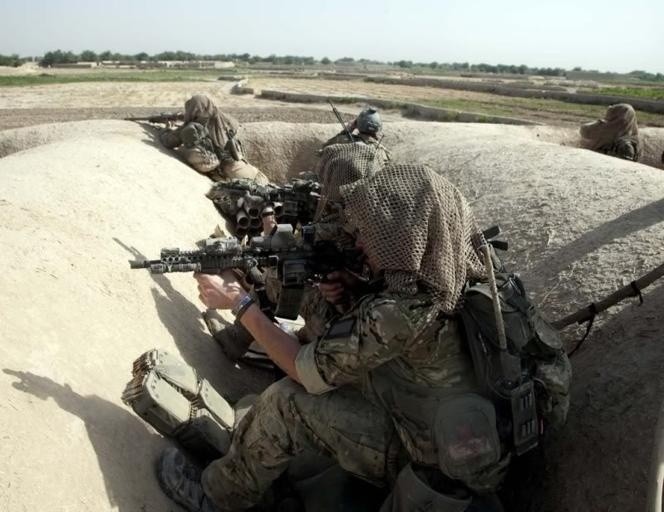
[463,268,573,446]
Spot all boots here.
[204,307,254,362]
[155,447,214,511]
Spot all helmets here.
[355,105,383,136]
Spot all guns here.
[130,221,369,320]
[124,113,184,127]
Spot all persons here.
[202,143,388,372]
[158,163,515,512]
[322,106,393,161]
[579,103,641,162]
[158,94,269,189]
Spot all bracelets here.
[231,293,252,315]
[236,299,256,321]
